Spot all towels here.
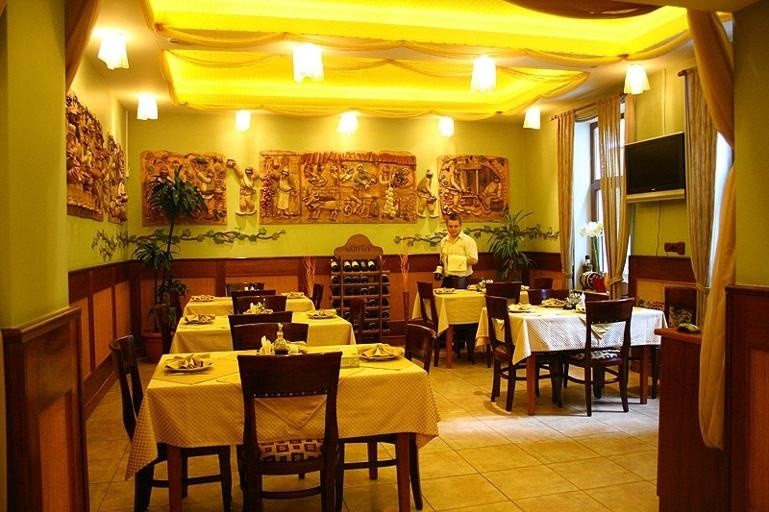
[165,356,204,370]
[360,343,399,358]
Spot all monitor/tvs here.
[623,130,685,204]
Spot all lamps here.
[522,105,544,131]
[135,90,159,121]
[624,63,651,96]
[293,44,326,84]
[96,23,130,69]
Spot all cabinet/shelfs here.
[654,323,728,512]
[329,234,391,343]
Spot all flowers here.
[579,221,604,274]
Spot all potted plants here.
[133,163,206,364]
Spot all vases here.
[592,272,607,293]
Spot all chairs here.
[149,278,356,358]
[109,334,233,511]
[338,324,438,511]
[236,350,347,511]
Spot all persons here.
[432,215,478,354]
[330,164,340,187]
[271,166,296,219]
[450,168,465,214]
[417,169,437,219]
[226,158,259,216]
[304,163,378,222]
[350,163,379,214]
[379,164,415,221]
[140,152,228,226]
[479,178,503,214]
[65,91,128,225]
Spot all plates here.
[312,315,333,319]
[540,304,565,308]
[362,353,399,361]
[509,309,533,313]
[169,362,214,373]
[189,320,211,324]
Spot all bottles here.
[434,256,445,280]
[329,259,340,272]
[331,275,389,330]
[360,261,368,271]
[343,261,351,271]
[274,332,287,354]
[368,260,377,272]
[351,260,360,272]
[583,255,593,272]
